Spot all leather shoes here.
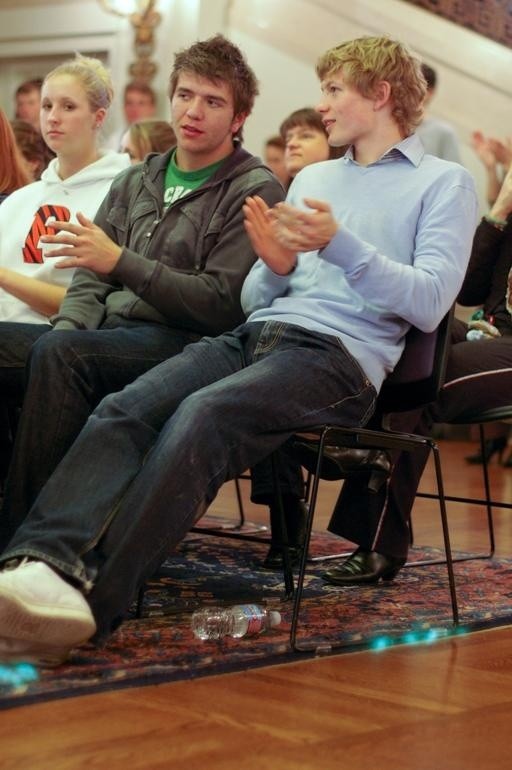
[263,501,310,568]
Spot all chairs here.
[135,267,460,656]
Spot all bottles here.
[192,604,285,639]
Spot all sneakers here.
[0,557,97,647]
[1,642,70,668]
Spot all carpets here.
[1,509,511,711]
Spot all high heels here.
[466,438,507,465]
[322,545,405,582]
[294,441,391,495]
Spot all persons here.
[0,30,512,665]
